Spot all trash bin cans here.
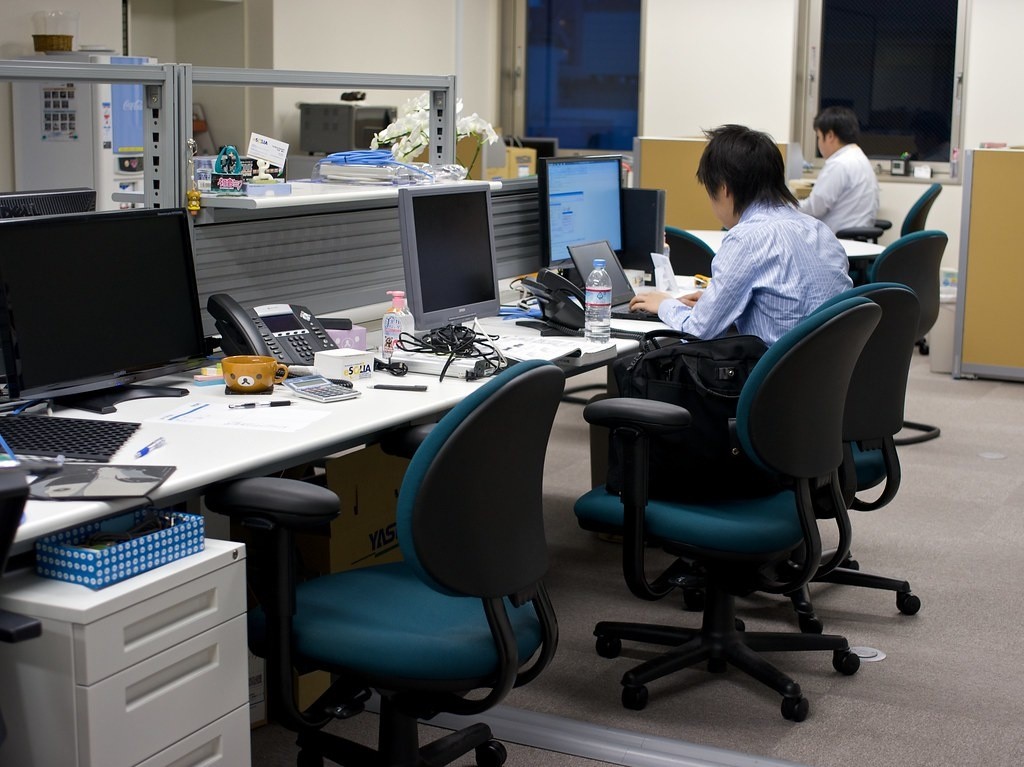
[927,286,958,375]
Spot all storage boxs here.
[411,127,508,181]
[34,505,204,590]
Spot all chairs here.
[575,185,948,722]
[203,359,566,767]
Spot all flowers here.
[370,95,498,178]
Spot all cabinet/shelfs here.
[0,540,251,767]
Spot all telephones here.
[207,294,339,378]
[521,268,585,331]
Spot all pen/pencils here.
[134,437,165,458]
[229,401,297,409]
[374,384,427,392]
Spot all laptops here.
[566,240,663,322]
[504,137,557,174]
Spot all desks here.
[0,230,892,724]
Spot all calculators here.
[282,374,362,403]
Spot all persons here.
[795,106,878,244]
[628,124,854,351]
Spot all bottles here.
[584,259,613,345]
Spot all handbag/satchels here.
[606,330,784,505]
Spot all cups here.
[220,355,288,391]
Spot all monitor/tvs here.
[0,187,210,414]
[537,154,625,291]
[399,182,501,343]
